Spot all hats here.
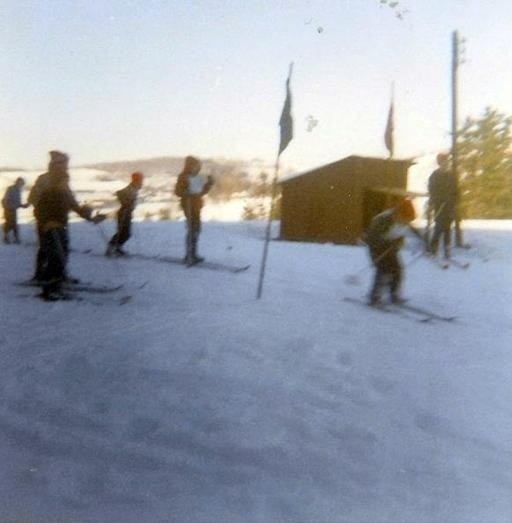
[437,152,449,160]
[48,150,69,164]
[398,199,416,220]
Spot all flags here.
[385,104,395,156]
[278,80,293,152]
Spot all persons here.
[27,151,105,301]
[362,210,409,308]
[107,172,143,257]
[427,154,461,260]
[175,156,214,267]
[2,178,30,244]
[30,175,80,286]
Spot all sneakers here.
[368,292,408,308]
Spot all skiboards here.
[10,277,149,307]
[69,248,94,256]
[428,251,470,272]
[97,251,161,261]
[163,254,252,274]
[343,292,456,324]
